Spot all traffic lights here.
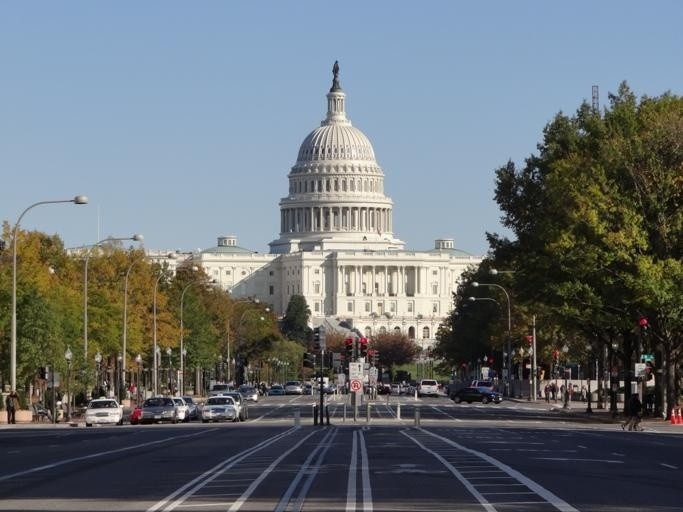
[527,335,532,345]
[369,351,374,361]
[360,337,367,358]
[375,351,380,360]
[38,365,48,381]
[345,338,354,358]
[302,353,314,368]
[313,327,321,350]
[639,317,648,340]
[552,350,559,367]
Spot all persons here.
[6,392,21,423]
[545,382,589,405]
[36,400,54,423]
[621,394,644,432]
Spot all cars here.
[448,387,504,405]
[80,396,125,427]
[127,391,249,424]
[209,377,415,402]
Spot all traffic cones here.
[676,407,682,424]
[669,408,676,424]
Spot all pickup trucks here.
[416,378,441,397]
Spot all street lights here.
[11,194,89,392]
[83,233,145,357]
[468,295,504,318]
[94,351,103,367]
[469,281,512,395]
[226,297,260,358]
[62,342,74,421]
[134,352,141,405]
[264,355,289,385]
[152,265,199,395]
[248,315,264,328]
[239,307,271,321]
[487,267,537,402]
[178,277,221,394]
[121,252,178,388]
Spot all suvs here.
[470,380,494,391]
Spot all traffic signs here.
[349,363,363,379]
[370,369,378,378]
